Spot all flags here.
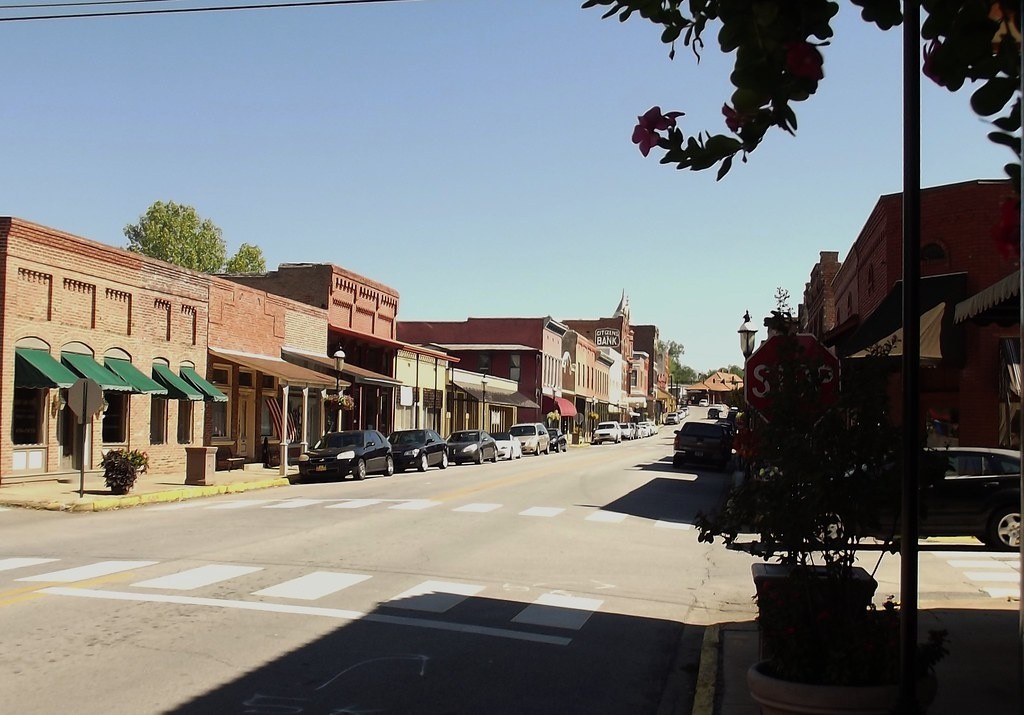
[266,397,297,443]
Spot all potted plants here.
[98,446,148,495]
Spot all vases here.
[747,661,939,715]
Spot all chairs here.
[215,447,245,472]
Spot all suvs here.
[593,421,622,446]
[665,412,681,425]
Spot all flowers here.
[695,319,982,694]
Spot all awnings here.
[542,395,577,416]
[61,352,133,393]
[152,365,204,401]
[953,268,1020,325]
[103,357,168,395]
[180,367,229,403]
[14,349,81,393]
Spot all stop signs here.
[744,331,843,436]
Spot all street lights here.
[481,377,488,432]
[591,395,597,440]
[552,387,559,427]
[332,347,346,433]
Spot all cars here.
[445,430,499,467]
[699,398,709,407]
[489,432,523,460]
[715,405,724,412]
[618,420,658,440]
[800,447,1024,552]
[546,427,568,454]
[674,407,690,419]
[507,423,551,455]
[385,428,449,472]
[298,430,395,483]
[672,421,736,473]
[707,408,719,419]
